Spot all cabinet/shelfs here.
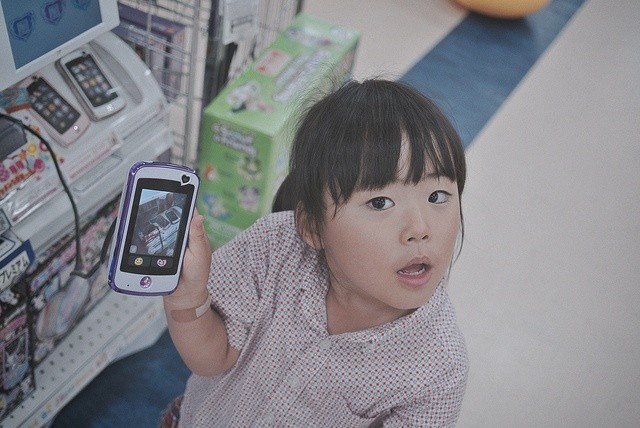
[0,0,206,427]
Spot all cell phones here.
[54,49,125,120]
[107,161,200,297]
[19,72,89,146]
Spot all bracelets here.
[166,293,212,322]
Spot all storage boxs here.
[190,9,363,253]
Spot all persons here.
[160,64,468,428]
